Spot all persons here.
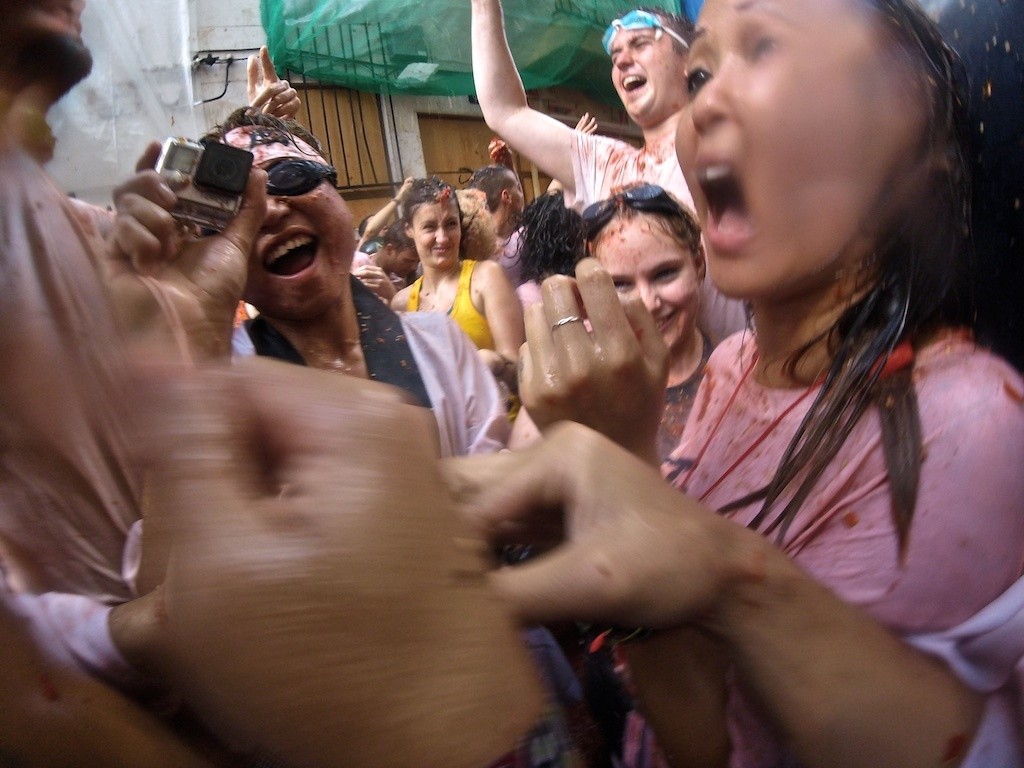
[510,182,723,478]
[2,144,591,768]
[352,104,597,420]
[438,422,1024,768]
[119,100,591,766]
[467,2,754,352]
[518,2,1022,768]
[0,0,304,704]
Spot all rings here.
[551,316,584,334]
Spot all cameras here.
[149,136,256,234]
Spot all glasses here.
[579,184,697,237]
[260,157,340,195]
[601,10,689,56]
